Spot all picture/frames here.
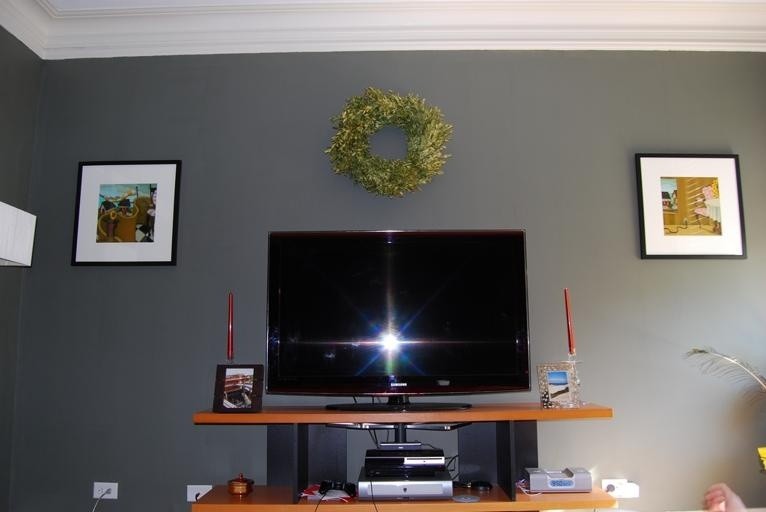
[636,153,746,259]
[70,161,182,265]
[539,363,581,409]
[213,364,263,414]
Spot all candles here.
[564,288,576,354]
[228,289,233,362]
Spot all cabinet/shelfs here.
[192,404,616,512]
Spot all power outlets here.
[603,479,639,499]
[186,485,211,502]
[94,482,118,499]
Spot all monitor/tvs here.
[264,228,532,412]
